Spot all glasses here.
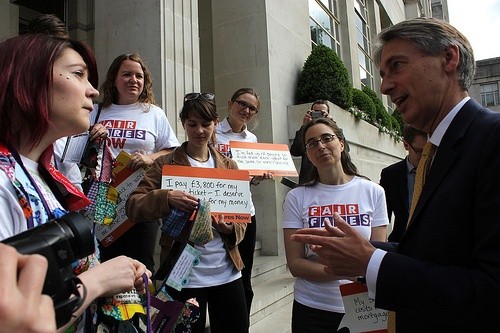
[234,99,256,111]
[408,142,422,157]
[305,135,335,150]
[184,92,216,106]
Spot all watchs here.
[355,275,367,286]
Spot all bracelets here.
[250,176,261,186]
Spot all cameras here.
[0,212,95,329]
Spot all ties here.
[386,141,438,333]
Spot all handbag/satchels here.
[80,142,119,226]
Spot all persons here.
[212,88,273,322]
[379,122,428,246]
[290,16,500,333]
[289,101,351,185]
[283,118,391,333]
[126,92,247,333]
[0,14,180,333]
